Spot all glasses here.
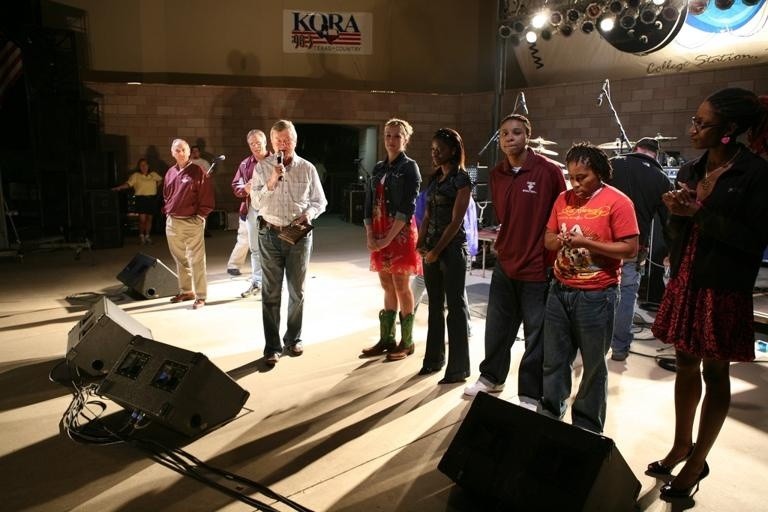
[498,0,763,44]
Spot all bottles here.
[637,138,659,154]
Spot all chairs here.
[241,284,262,297]
[227,269,242,275]
[464,379,504,395]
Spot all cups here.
[690,117,722,132]
[264,221,287,233]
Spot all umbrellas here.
[418,367,432,376]
[193,299,205,310]
[267,353,278,364]
[171,293,195,302]
[290,344,303,355]
[439,377,451,385]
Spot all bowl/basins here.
[576,198,593,221]
[701,150,741,189]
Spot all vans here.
[660,460,709,497]
[647,444,696,473]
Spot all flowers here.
[276,151,285,182]
[520,92,529,115]
[212,155,226,162]
[596,81,607,106]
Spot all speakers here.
[386,312,414,361]
[362,310,398,355]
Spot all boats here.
[90,189,121,249]
[66,296,153,377]
[117,252,179,299]
[437,392,642,512]
[99,335,250,437]
[345,190,366,223]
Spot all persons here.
[536,143,641,434]
[231,129,273,302]
[112,158,164,244]
[647,87,768,499]
[362,118,424,361]
[311,158,327,184]
[161,138,216,309]
[227,197,251,276]
[396,190,479,341]
[250,120,328,364]
[189,145,215,178]
[599,137,676,361]
[415,127,472,384]
[464,114,567,411]
